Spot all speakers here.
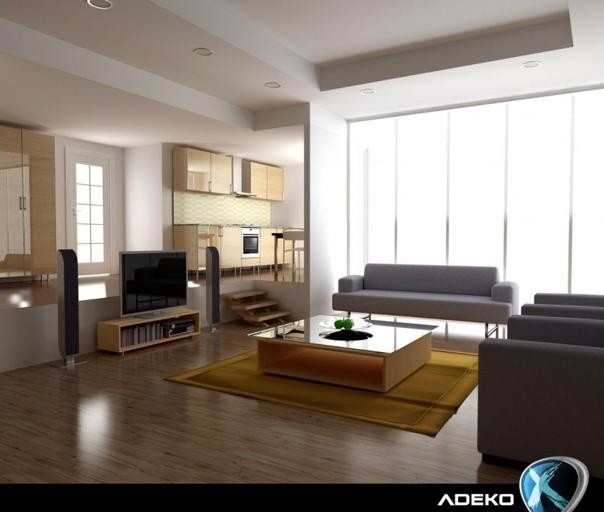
[205,245,220,334]
[56,247,79,371]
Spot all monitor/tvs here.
[119,250,188,320]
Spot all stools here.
[282,227,304,283]
[272,233,283,282]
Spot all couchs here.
[478,314,604,479]
[332,264,519,340]
[521,292,604,315]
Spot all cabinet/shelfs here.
[260,228,277,273]
[97,309,201,354]
[241,160,283,201]
[277,228,295,275]
[174,224,220,279]
[173,147,231,195]
[242,226,260,276]
[0,125,57,288]
[220,226,242,277]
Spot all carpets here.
[164,345,478,439]
